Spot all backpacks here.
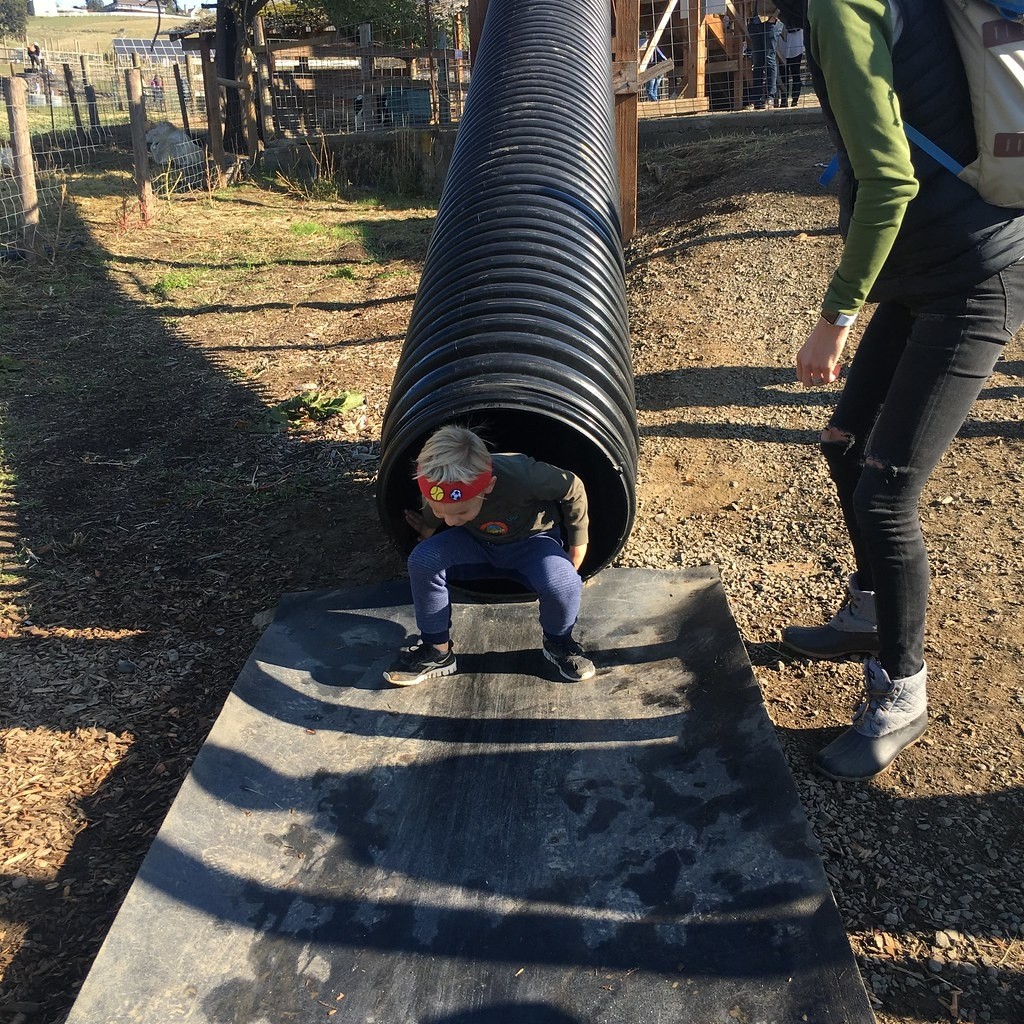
[895,3,1024,209]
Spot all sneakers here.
[542,632,595,682]
[382,641,458,687]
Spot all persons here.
[28,42,40,72]
[783,0,1024,781]
[748,15,787,108]
[151,74,166,112]
[383,426,596,686]
[142,75,149,109]
[647,75,662,102]
[778,25,803,108]
[180,74,194,114]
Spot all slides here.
[375,0,639,603]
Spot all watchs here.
[819,308,858,325]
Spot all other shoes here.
[760,99,773,109]
[791,101,797,107]
[780,102,788,107]
[745,100,762,110]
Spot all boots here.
[812,660,929,785]
[783,575,881,658]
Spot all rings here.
[813,378,822,384]
